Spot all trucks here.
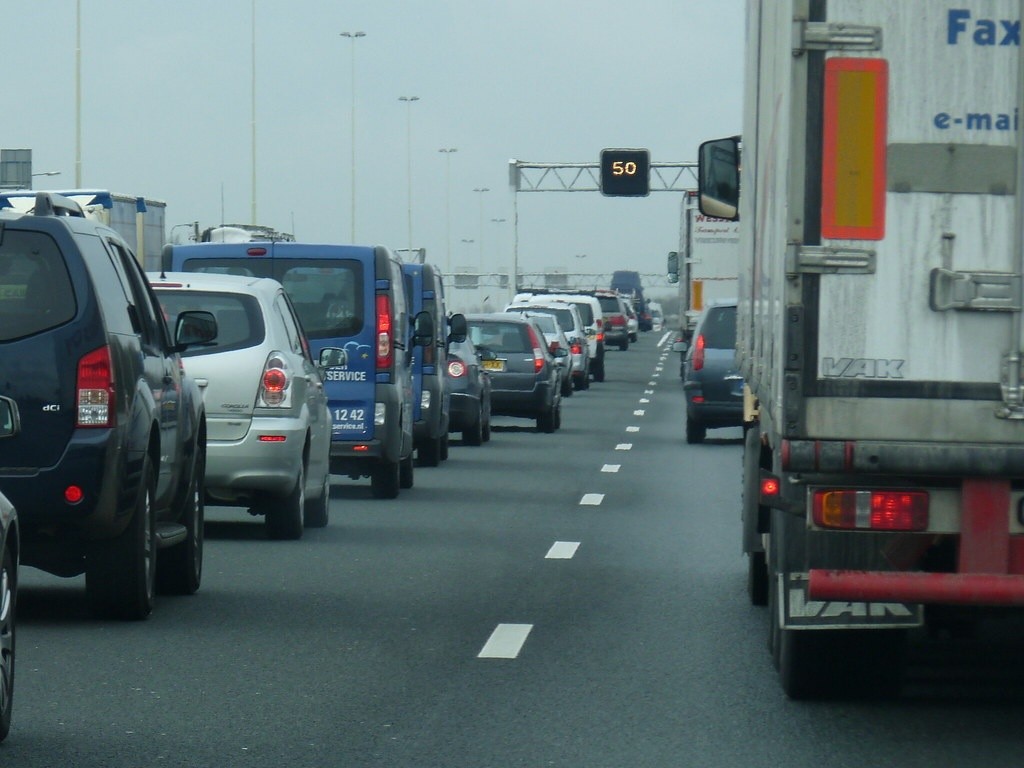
[693,0,1024,702]
[667,188,740,380]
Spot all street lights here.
[438,147,460,273]
[473,187,490,276]
[398,96,420,264]
[340,31,367,247]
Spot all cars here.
[446,320,496,446]
[142,271,349,540]
[669,302,778,441]
[448,309,564,434]
[477,287,665,396]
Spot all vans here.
[160,227,435,497]
[282,249,469,498]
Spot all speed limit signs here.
[599,148,649,196]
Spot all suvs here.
[0,188,218,627]
